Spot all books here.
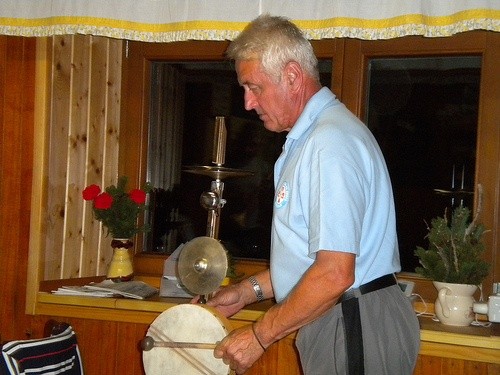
[51,279,160,299]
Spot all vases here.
[106,238,135,283]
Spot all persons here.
[192,13,420,375]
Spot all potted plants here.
[414,205,490,327]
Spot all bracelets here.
[251,321,267,351]
[249,275,265,303]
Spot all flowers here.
[82,176,154,239]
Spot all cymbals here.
[177,236,228,296]
[180,164,258,184]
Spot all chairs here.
[0,319,84,375]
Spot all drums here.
[140,300,237,375]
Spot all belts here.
[335,273,397,305]
